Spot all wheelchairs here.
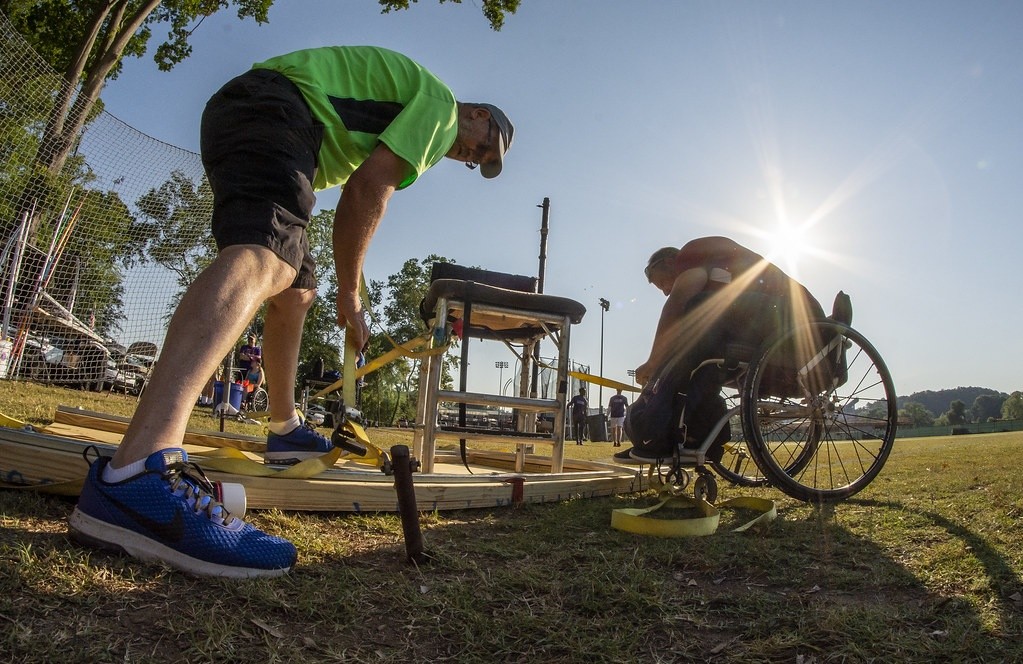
[666,290,898,504]
[241,388,269,412]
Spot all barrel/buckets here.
[213,381,244,413]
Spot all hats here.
[248,332,256,340]
[250,356,262,364]
[478,103,516,177]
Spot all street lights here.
[599,298,610,414]
[495,361,508,419]
[627,370,636,404]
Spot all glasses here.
[645,256,665,278]
[467,120,494,168]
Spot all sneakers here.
[612,447,660,469]
[69,444,298,584]
[265,418,335,471]
[631,441,705,467]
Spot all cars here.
[28,337,136,390]
[110,350,153,396]
[295,403,325,426]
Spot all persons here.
[567,388,589,445]
[613,236,833,465]
[355,342,370,404]
[240,356,264,399]
[68,44,516,580]
[240,333,261,380]
[606,389,628,447]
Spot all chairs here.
[413,260,585,474]
[299,376,339,419]
